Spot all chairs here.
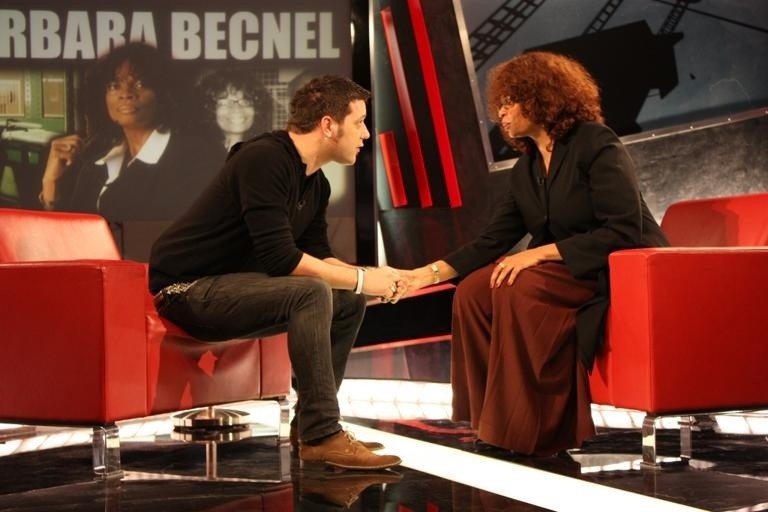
[0,207,295,478]
[587,192,766,474]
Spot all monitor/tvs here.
[0,0,378,265]
[451,0,768,174]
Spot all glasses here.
[216,98,254,109]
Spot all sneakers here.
[288,425,402,471]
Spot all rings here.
[498,261,504,268]
[390,284,396,294]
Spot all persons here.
[388,50,671,455]
[145,71,400,469]
[36,40,206,218]
[191,66,272,153]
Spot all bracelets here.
[430,263,440,285]
[38,190,61,205]
[353,267,365,295]
